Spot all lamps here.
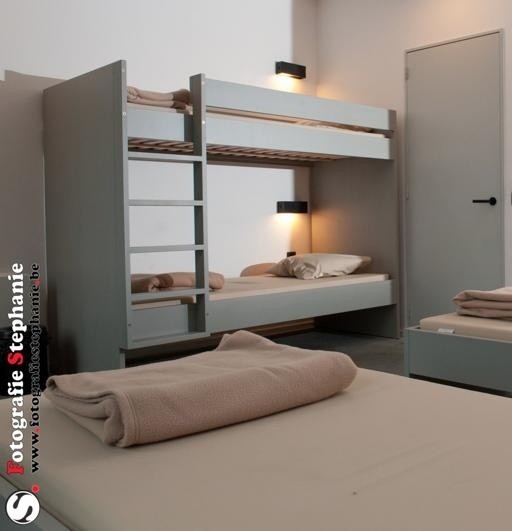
[272,60,309,83]
[276,200,310,216]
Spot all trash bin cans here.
[0,324,51,398]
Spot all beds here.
[404,285,511,395]
[44,58,401,382]
[1,329,511,531]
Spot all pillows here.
[263,252,372,280]
